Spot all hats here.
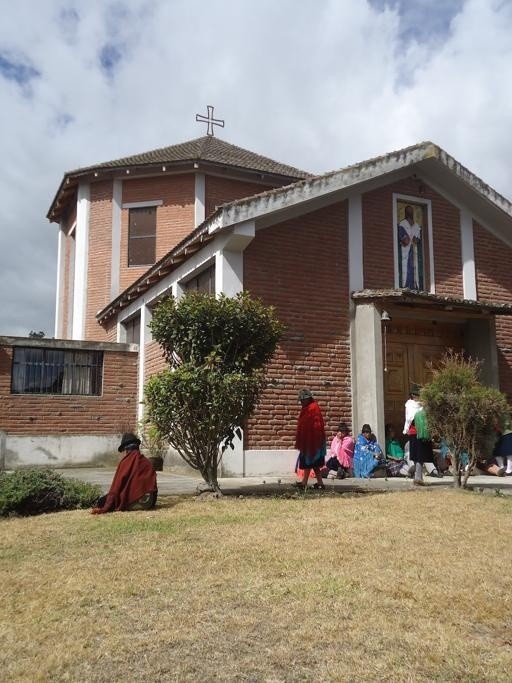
[338,423,394,433]
[409,383,423,395]
[297,387,313,399]
[118,433,141,451]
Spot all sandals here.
[429,468,441,477]
[413,479,428,486]
[291,481,325,490]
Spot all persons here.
[403,390,442,478]
[90,430,159,513]
[434,415,512,477]
[353,423,381,479]
[291,389,326,489]
[402,407,444,485]
[385,422,411,478]
[397,204,420,287]
[322,421,353,478]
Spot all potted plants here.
[137,419,168,471]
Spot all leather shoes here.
[497,467,512,477]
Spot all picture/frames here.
[392,192,436,295]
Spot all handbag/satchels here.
[407,423,417,437]
